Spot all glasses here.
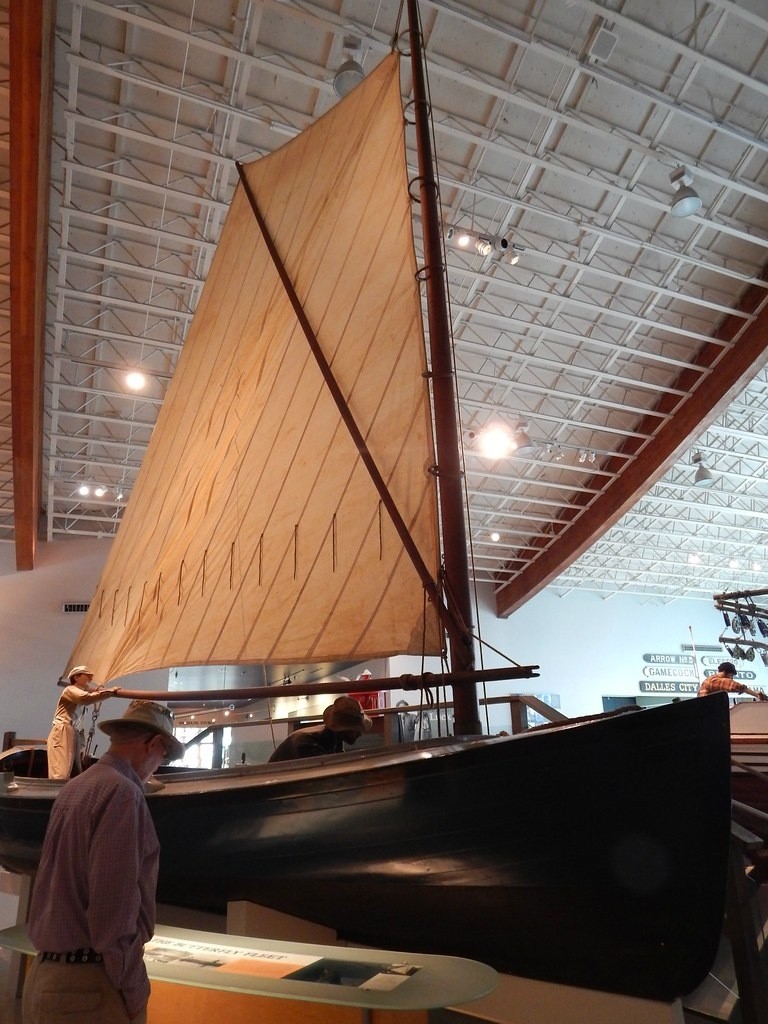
[144,734,172,759]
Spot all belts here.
[37,949,104,964]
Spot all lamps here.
[473,233,491,256]
[493,237,508,252]
[514,422,536,456]
[75,479,123,499]
[506,242,519,265]
[667,164,702,219]
[691,452,714,487]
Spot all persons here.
[46,666,121,779]
[396,700,420,742]
[267,695,373,763]
[21,700,186,1024]
[697,662,768,703]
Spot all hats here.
[68,665,95,679]
[323,697,373,733]
[98,698,185,762]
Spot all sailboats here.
[0,0,768,1024]
